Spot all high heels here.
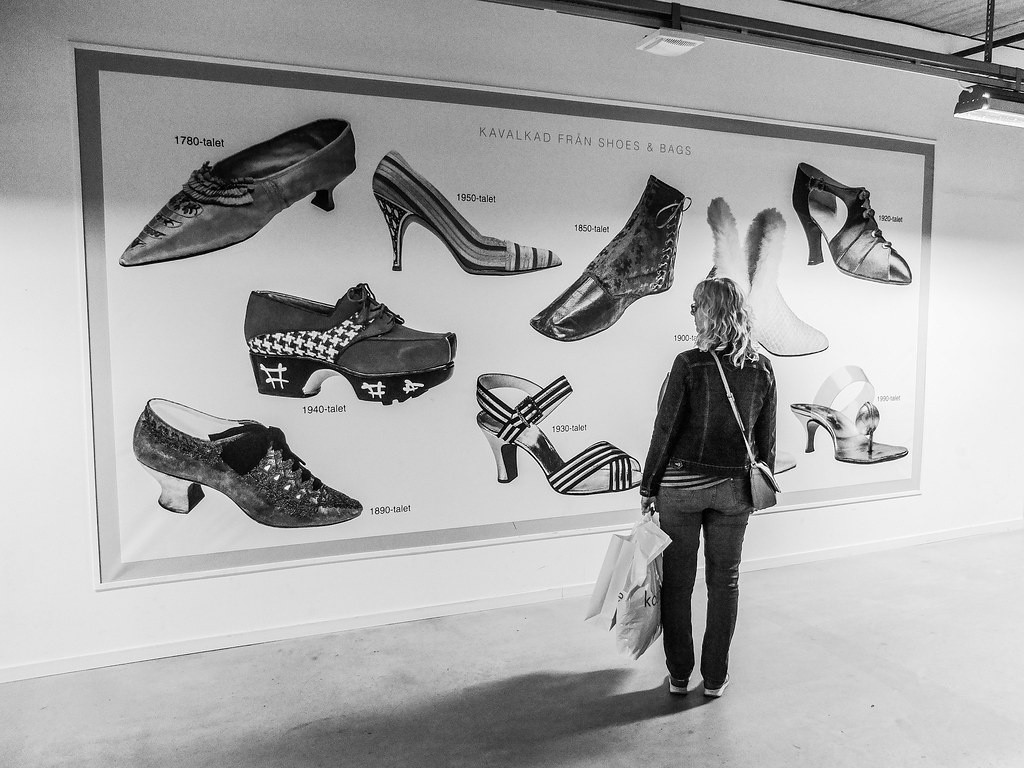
[475,374,644,495]
[791,162,912,284]
[118,118,356,268]
[372,150,563,277]
[244,287,458,405]
[790,368,907,465]
[132,399,359,527]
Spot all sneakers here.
[668,674,688,693]
[705,673,730,696]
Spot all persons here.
[640,277,777,696]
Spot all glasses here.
[690,303,697,315]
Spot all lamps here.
[954,83,1024,128]
[636,4,704,58]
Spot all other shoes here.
[531,174,692,342]
[708,198,828,358]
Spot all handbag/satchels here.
[584,510,674,662]
[748,461,782,509]
